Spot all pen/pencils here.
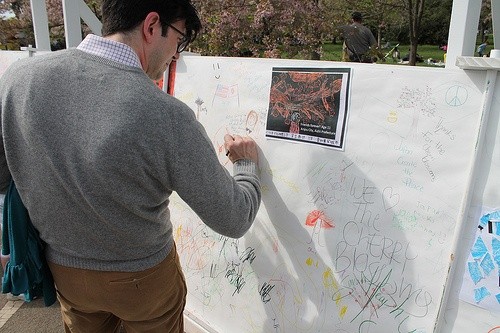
[225,137,235,157]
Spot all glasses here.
[160,19,190,53]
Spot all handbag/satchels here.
[361,55,374,63]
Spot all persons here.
[341,12,386,63]
[0,0,262,333]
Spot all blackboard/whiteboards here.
[157,50,500,333]
